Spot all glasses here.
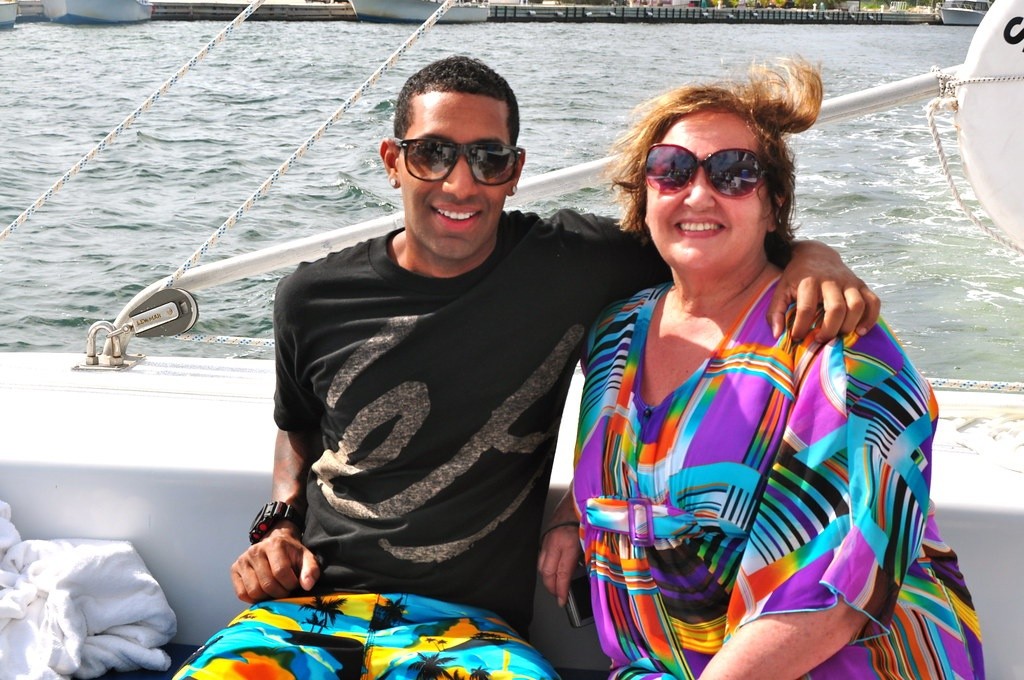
[643,142,776,200]
[393,137,526,186]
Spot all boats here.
[348,0,490,24]
[41,0,153,26]
[937,0,991,26]
[0,1,18,28]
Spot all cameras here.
[565,575,595,628]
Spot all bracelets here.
[540,521,579,546]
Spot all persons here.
[538,55,985,680]
[171,56,880,680]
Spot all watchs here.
[249,500,306,544]
